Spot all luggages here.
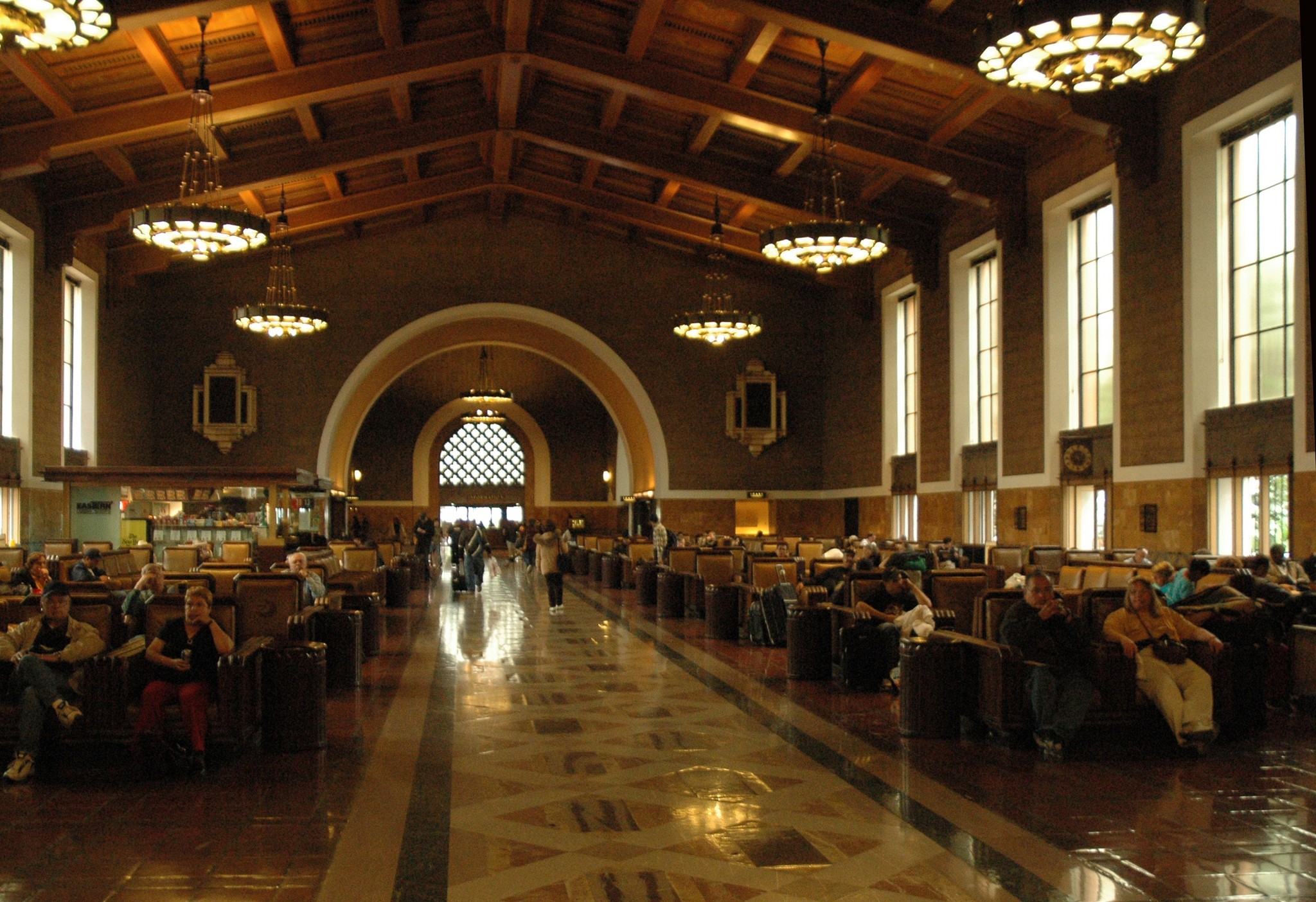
[759,563,798,646]
[452,559,467,592]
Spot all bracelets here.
[206,618,214,626]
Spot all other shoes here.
[55,700,85,730]
[1034,732,1064,758]
[3,752,34,781]
[527,565,532,573]
[879,676,893,691]
[550,603,563,610]
[191,749,208,777]
[1178,722,1221,759]
[476,585,482,592]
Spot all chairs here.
[572,531,1316,741]
[0,538,418,775]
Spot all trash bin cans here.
[787,605,830,679]
[315,609,363,688]
[342,592,379,656]
[402,553,420,588]
[636,566,657,608]
[899,637,960,738]
[602,552,620,588]
[705,584,739,639]
[261,640,327,749]
[657,572,683,618]
[564,548,601,581]
[386,566,409,608]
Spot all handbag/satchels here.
[488,556,502,578]
[557,553,572,575]
[1152,634,1188,663]
[903,555,926,570]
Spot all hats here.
[880,566,903,580]
[86,547,103,561]
[41,580,69,597]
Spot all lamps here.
[129,13,272,263]
[760,34,893,273]
[459,347,514,423]
[672,192,765,348]
[972,0,1209,93]
[231,183,330,338]
[0,0,120,59]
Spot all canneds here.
[181,649,191,665]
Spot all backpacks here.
[750,602,770,645]
[842,616,900,695]
[665,529,677,548]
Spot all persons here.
[612,533,633,590]
[998,570,1093,758]
[649,514,668,565]
[846,532,915,568]
[1150,543,1312,656]
[796,547,875,634]
[411,512,436,580]
[69,548,126,622]
[430,518,444,567]
[935,537,958,570]
[1101,577,1225,758]
[447,518,492,594]
[120,563,187,641]
[0,581,105,782]
[854,567,936,693]
[8,552,53,596]
[502,517,545,574]
[388,516,406,544]
[534,519,569,618]
[281,551,326,607]
[755,530,764,537]
[1122,546,1153,566]
[365,540,385,567]
[136,585,235,772]
[676,529,746,549]
[768,541,790,557]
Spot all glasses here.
[37,561,46,565]
